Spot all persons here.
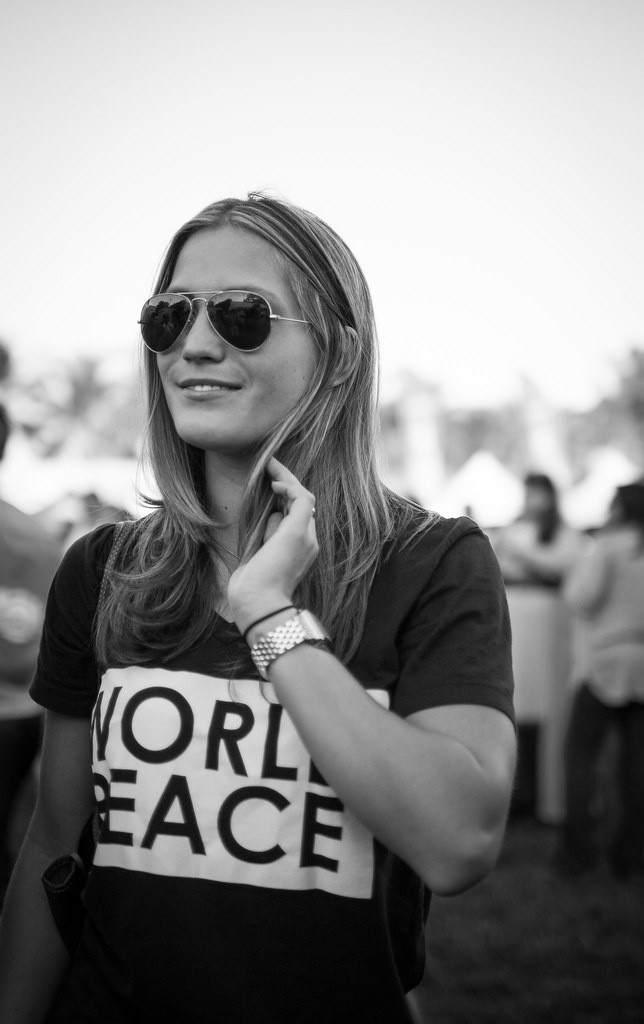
[495,471,643,869]
[0,193,519,1023]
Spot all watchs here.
[251,609,331,681]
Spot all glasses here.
[138,288,312,354]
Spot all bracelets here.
[242,602,297,642]
